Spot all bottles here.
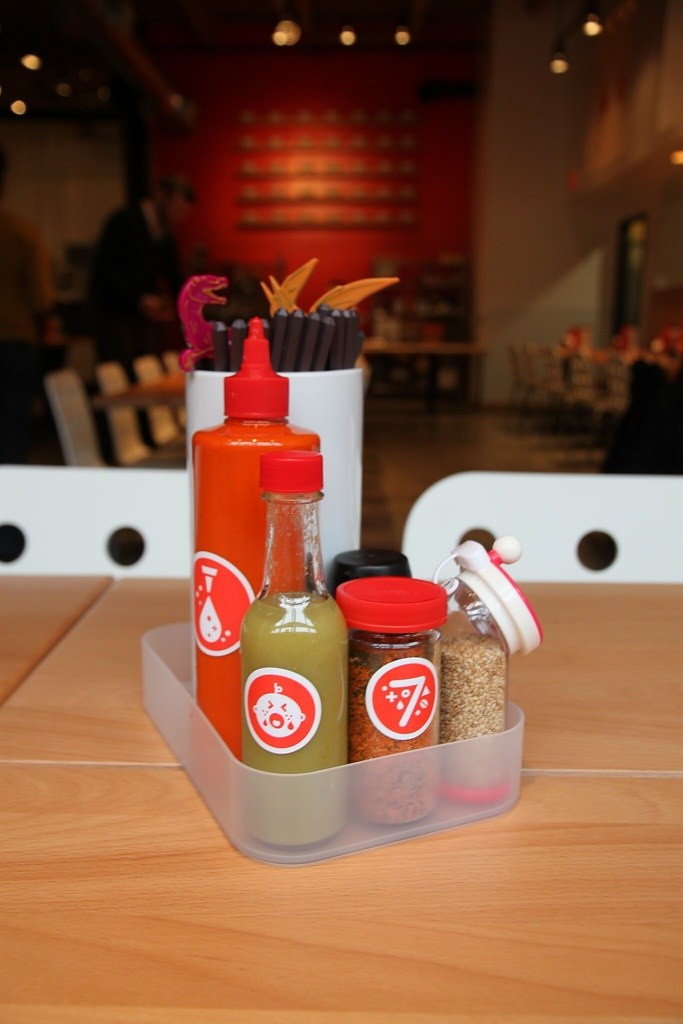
[335,578,448,827]
[432,537,543,807]
[191,316,322,763]
[333,548,413,592]
[239,449,349,850]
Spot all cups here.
[183,369,365,596]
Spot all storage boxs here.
[140,622,525,863]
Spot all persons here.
[0,148,53,461]
[86,175,196,360]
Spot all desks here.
[0,573,683,1024]
[363,339,488,414]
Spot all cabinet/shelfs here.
[368,254,474,398]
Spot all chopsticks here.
[213,305,360,373]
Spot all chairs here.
[42,351,185,469]
[507,341,630,446]
[400,470,683,584]
[0,463,191,580]
